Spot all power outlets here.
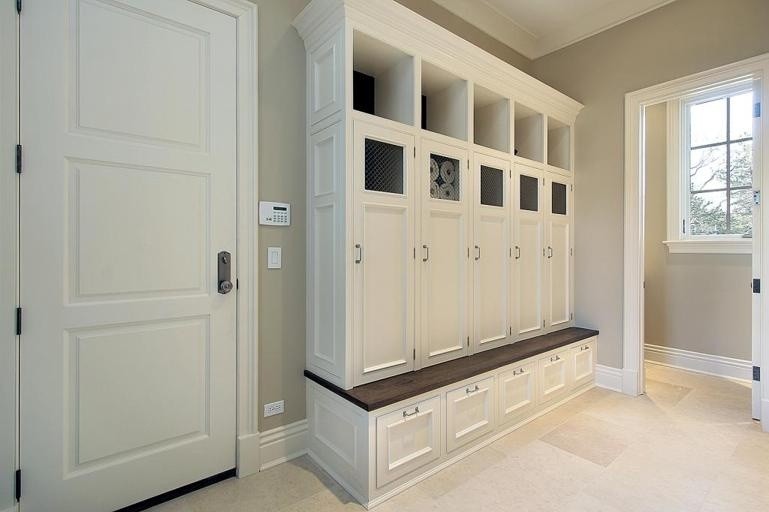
[263,400,286,417]
